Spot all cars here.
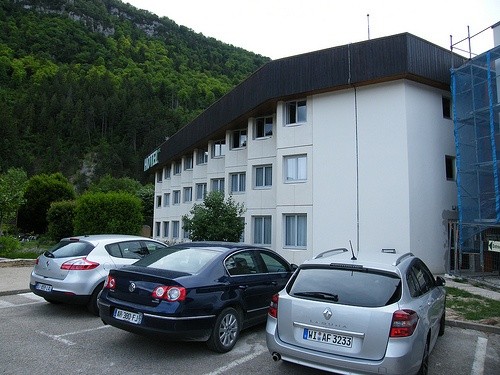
[97,241,299,354]
[265,240,447,375]
[29,234,169,316]
